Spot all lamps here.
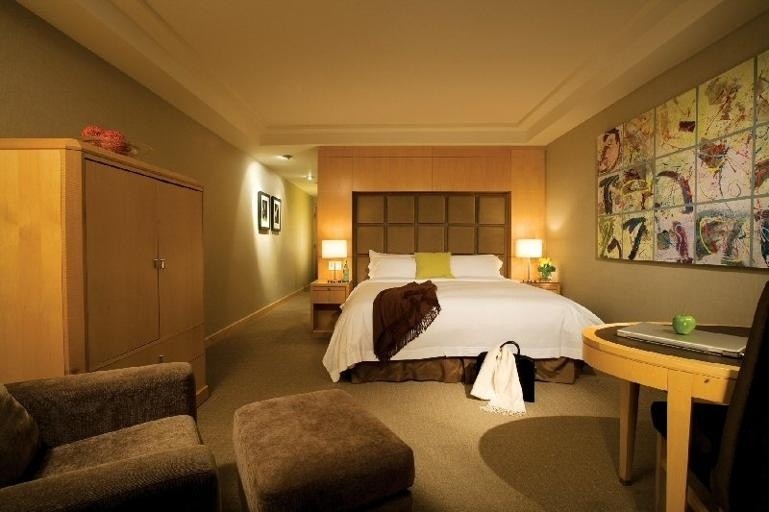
[516,238,542,281]
[321,239,348,283]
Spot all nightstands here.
[522,278,560,296]
[309,277,353,340]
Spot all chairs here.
[0,361,221,510]
[649,280,769,512]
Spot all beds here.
[333,274,592,386]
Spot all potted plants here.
[537,257,557,280]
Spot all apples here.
[672,313,696,335]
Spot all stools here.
[230,387,415,511]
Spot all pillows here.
[367,247,504,280]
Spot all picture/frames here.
[257,190,283,231]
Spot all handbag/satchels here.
[476,341,535,402]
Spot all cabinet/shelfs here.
[1,136,212,413]
